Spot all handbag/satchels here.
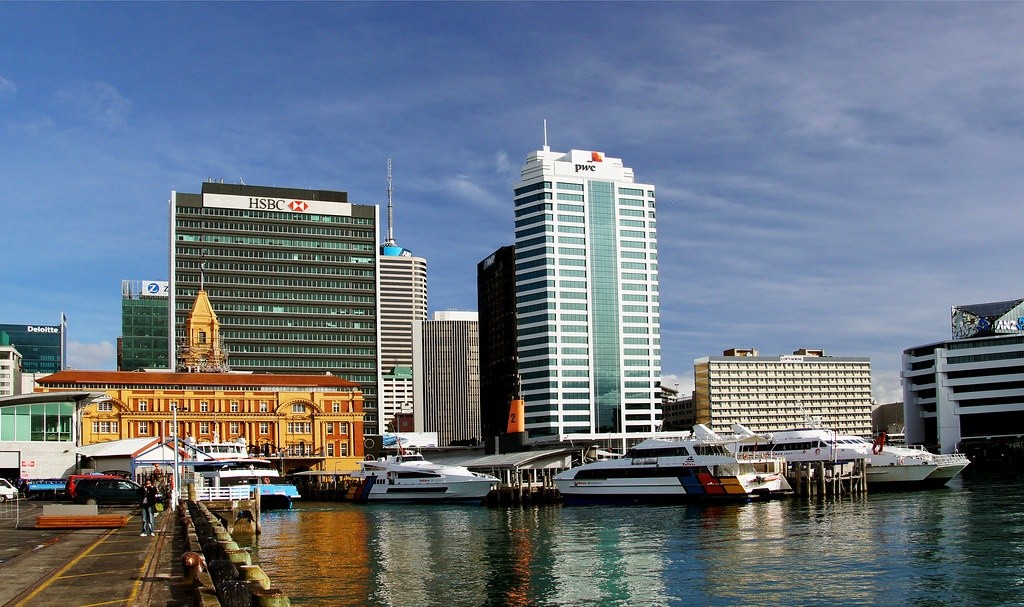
[155,502,164,512]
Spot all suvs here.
[66,475,124,501]
[74,479,165,507]
[0,478,19,503]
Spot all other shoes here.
[140,532,148,536]
[149,532,155,537]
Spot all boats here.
[724,429,971,489]
[189,440,302,511]
[551,423,795,500]
[349,453,501,506]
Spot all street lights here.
[173,404,188,510]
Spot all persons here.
[153,464,160,482]
[138,480,158,537]
[7,477,31,499]
